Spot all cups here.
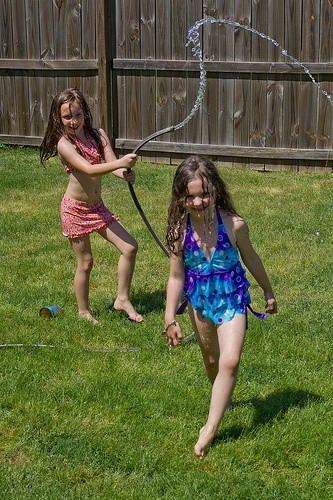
[39,305,62,318]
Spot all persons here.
[164,156,278,461]
[38,88,144,325]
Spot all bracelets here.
[163,320,177,333]
